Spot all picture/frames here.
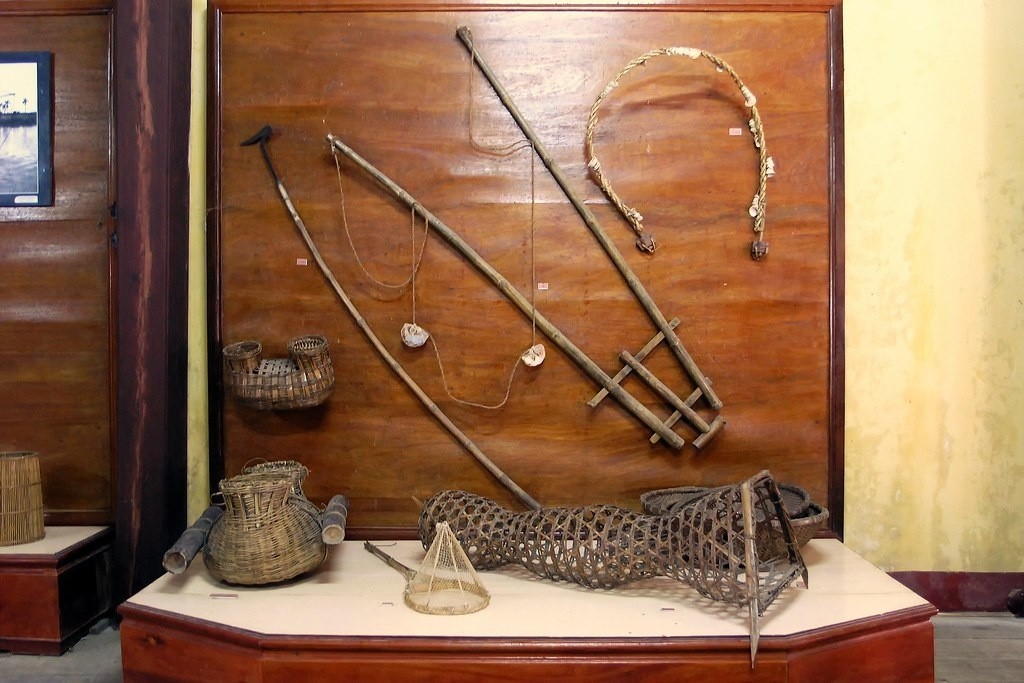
[0,51,55,207]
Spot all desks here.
[119,540,939,683]
[0,526,113,657]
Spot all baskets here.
[641,482,831,565]
